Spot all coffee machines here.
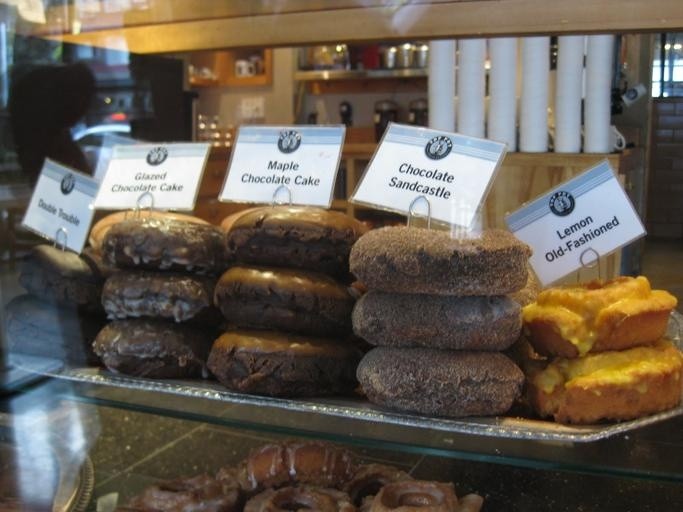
[550,33,631,155]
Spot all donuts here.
[5,204,532,416]
[121,440,486,512]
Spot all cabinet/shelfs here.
[294,69,427,154]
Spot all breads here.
[521,276,682,422]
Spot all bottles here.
[196,110,235,148]
[372,97,401,143]
[408,99,427,127]
[377,41,428,68]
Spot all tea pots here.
[608,125,625,152]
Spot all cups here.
[233,53,265,77]
[620,84,647,108]
